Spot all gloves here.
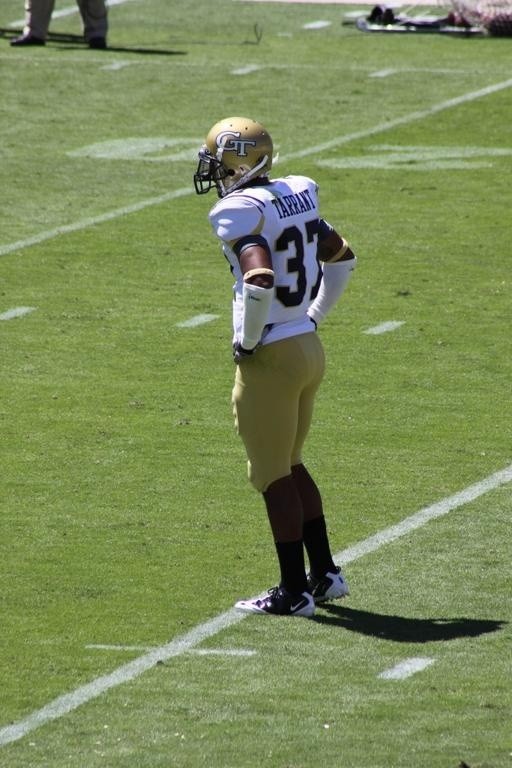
[233,338,263,364]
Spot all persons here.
[8,0,110,49]
[193,118,356,618]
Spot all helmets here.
[192,116,276,199]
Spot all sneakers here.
[305,564,350,605]
[233,584,316,617]
[85,37,108,51]
[8,31,47,47]
